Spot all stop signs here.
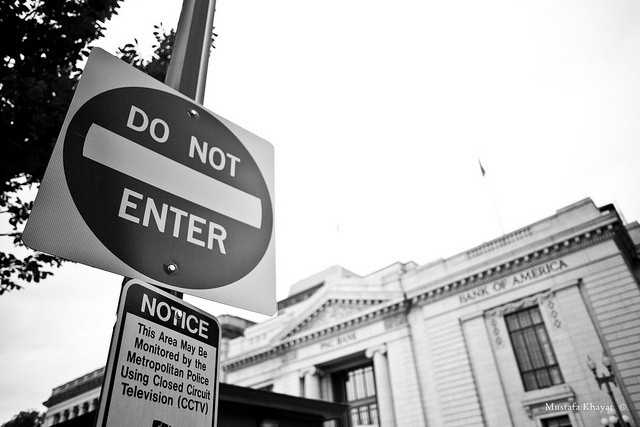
[23,48,277,316]
